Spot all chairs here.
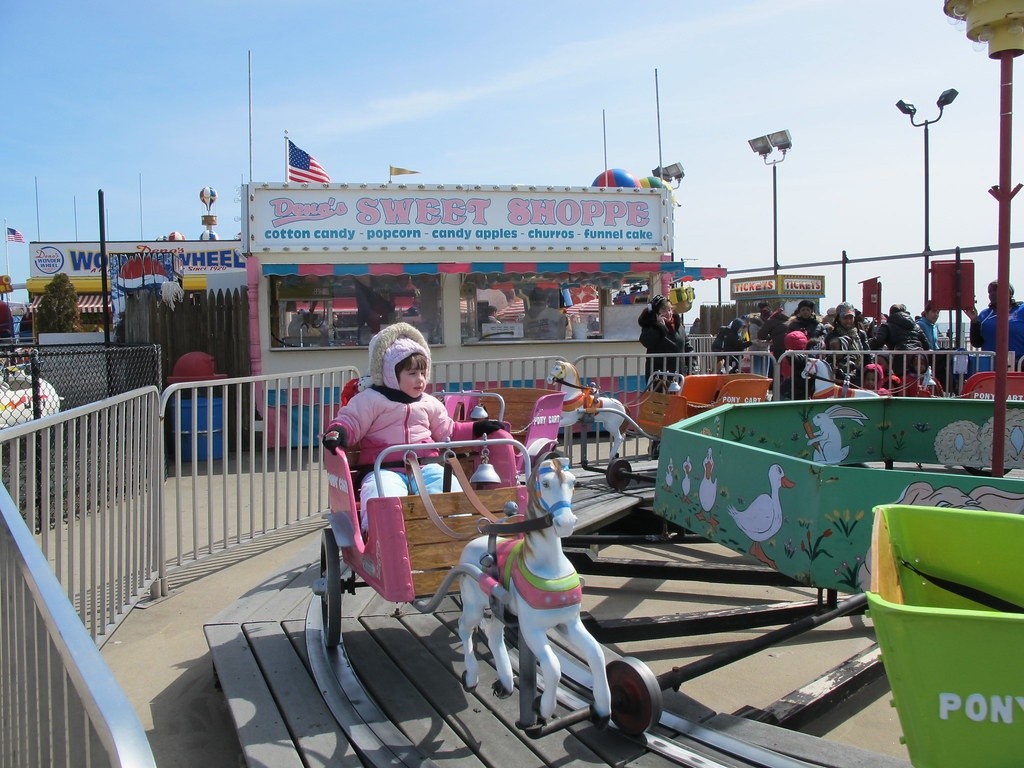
[681,373,773,406]
[963,372,1024,401]
[443,388,567,470]
[866,503,1024,768]
[323,429,517,551]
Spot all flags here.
[288,140,330,183]
[7,227,25,243]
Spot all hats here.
[836,302,856,317]
[806,339,821,349]
[650,294,666,309]
[381,336,428,390]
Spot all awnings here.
[27,293,112,313]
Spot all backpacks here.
[711,327,738,351]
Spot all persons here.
[637,295,698,453]
[322,322,506,540]
[489,305,501,324]
[748,303,789,378]
[961,280,1024,372]
[712,318,753,372]
[781,300,944,397]
[523,288,568,340]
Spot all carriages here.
[310,388,614,734]
[545,359,773,467]
[800,355,1024,404]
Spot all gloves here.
[323,426,348,455]
[474,420,505,438]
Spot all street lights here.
[894,88,959,309]
[747,129,793,275]
